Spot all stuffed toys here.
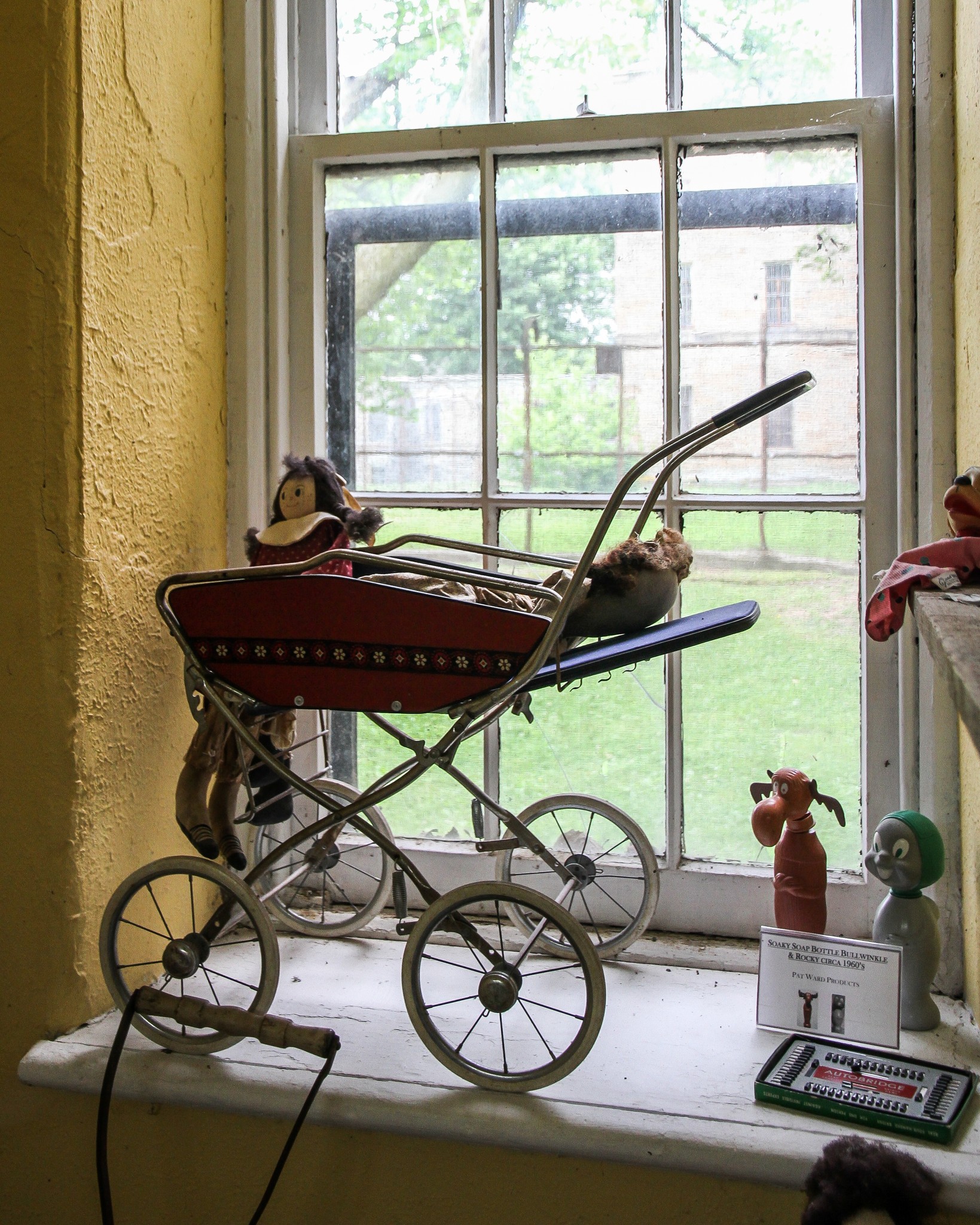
[562,528,692,639]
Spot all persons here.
[174,456,381,863]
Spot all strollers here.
[96,368,816,1095]
